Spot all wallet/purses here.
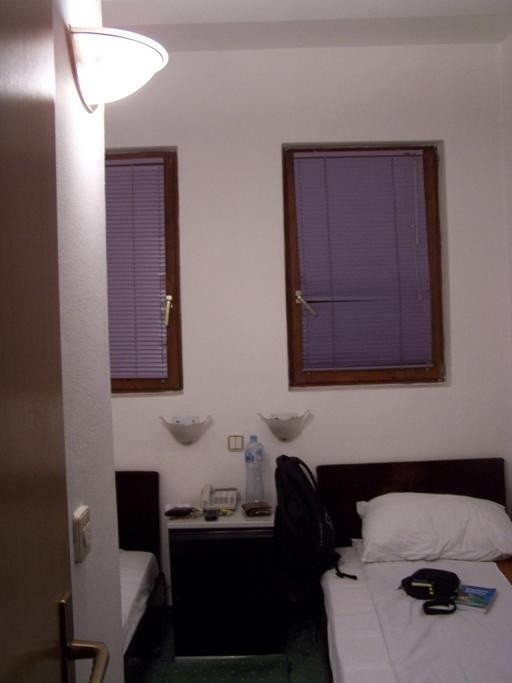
[241,500,272,516]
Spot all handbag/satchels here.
[403,564,462,615]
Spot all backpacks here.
[278,451,339,569]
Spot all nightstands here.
[167,508,287,664]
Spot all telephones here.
[199,484,239,510]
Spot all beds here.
[115,470,167,682]
[316,457,512,683]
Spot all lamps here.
[158,414,211,446]
[64,27,169,114]
[257,408,309,442]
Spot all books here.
[449,584,498,614]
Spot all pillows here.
[357,492,512,565]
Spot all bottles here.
[243,434,265,505]
